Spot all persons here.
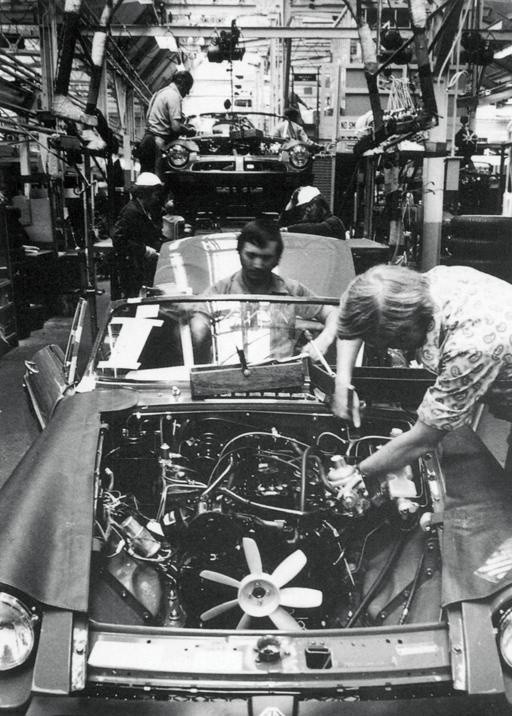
[269,106,325,157]
[326,262,512,505]
[110,170,173,301]
[137,69,197,176]
[279,184,346,242]
[190,220,341,367]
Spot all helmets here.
[291,185,322,206]
[134,171,164,187]
